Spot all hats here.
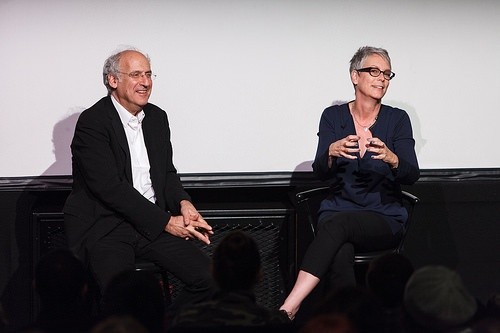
[403,266,477,329]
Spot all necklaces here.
[352,102,377,131]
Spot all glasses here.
[356,67,395,80]
[117,71,157,80]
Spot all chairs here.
[133,256,172,310]
[295,186,421,280]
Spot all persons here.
[62,49,214,333]
[0,230,500,333]
[274,47,420,333]
